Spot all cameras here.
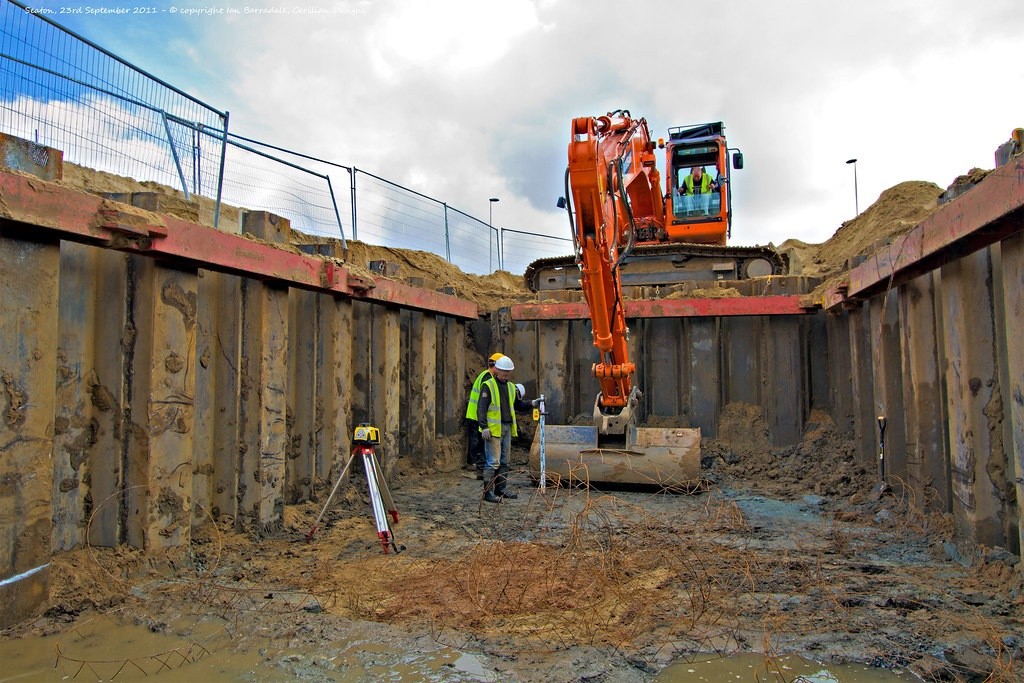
[354,423,381,444]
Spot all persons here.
[676,167,715,215]
[464,351,526,478]
[476,356,547,503]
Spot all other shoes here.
[466,461,476,470]
[476,474,484,481]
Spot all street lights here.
[490,197,499,274]
[847,159,859,216]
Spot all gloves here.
[532,398,547,408]
[482,429,492,441]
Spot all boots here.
[484,481,500,503]
[493,474,517,499]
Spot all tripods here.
[305,445,399,554]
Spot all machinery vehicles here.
[524,110,786,491]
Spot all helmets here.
[495,356,514,370]
[515,383,525,400]
[489,353,504,361]
